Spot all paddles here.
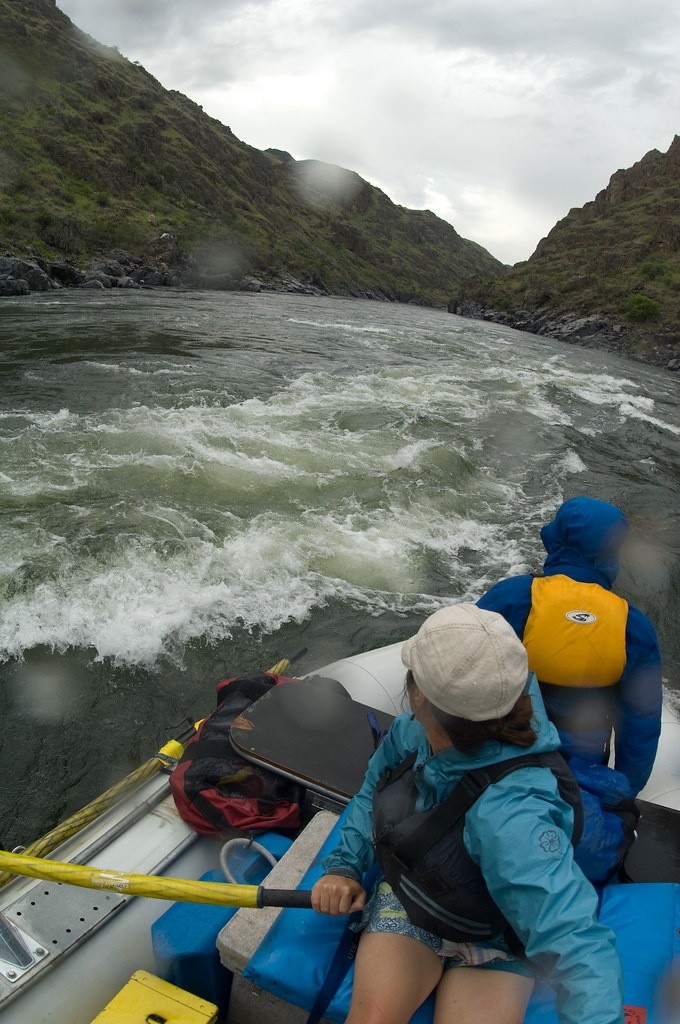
[0,640,319,896]
[0,821,373,918]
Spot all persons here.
[313,601,625,1023]
[475,497,666,920]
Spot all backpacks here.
[169,672,314,841]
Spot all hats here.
[401,603,529,721]
